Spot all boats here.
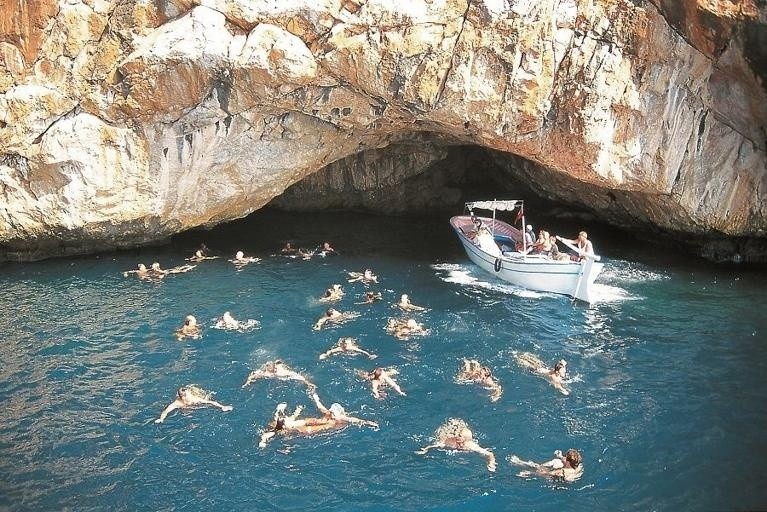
[446,193,608,303]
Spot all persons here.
[507,449,584,484]
[411,414,498,474]
[258,269,433,451]
[467,215,595,263]
[515,350,571,396]
[154,385,233,426]
[120,240,341,282]
[453,356,503,403]
[173,314,201,342]
[212,310,259,333]
[239,358,320,391]
